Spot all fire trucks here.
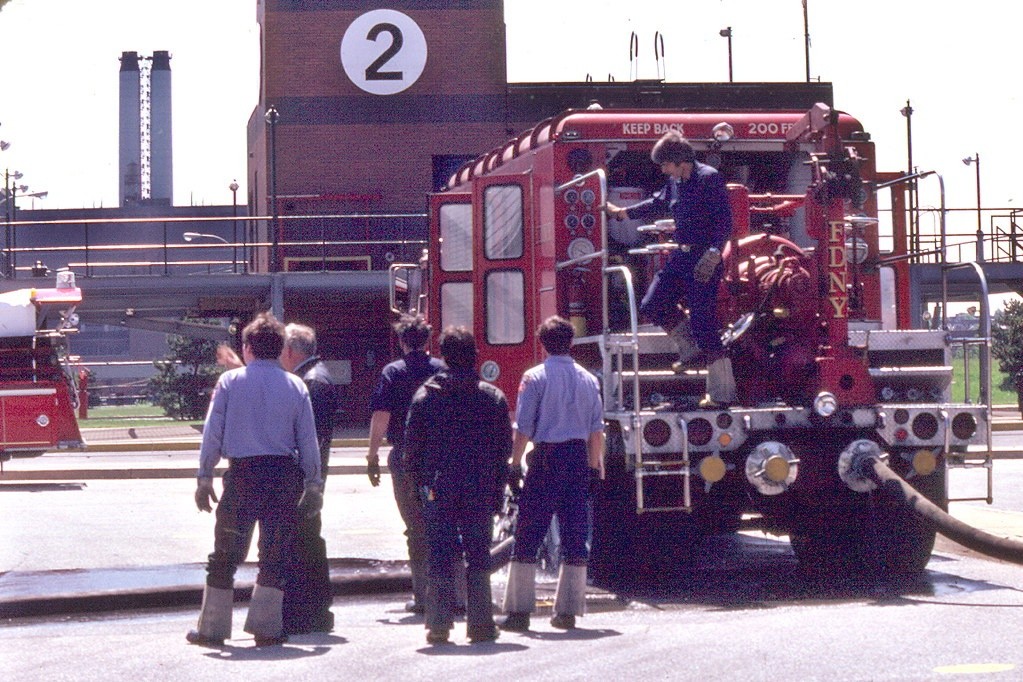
[0,269,88,465]
[385,94,997,593]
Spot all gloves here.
[595,202,624,222]
[366,454,381,486]
[692,250,721,283]
[296,482,324,519]
[195,477,217,513]
[506,463,525,496]
[584,467,603,496]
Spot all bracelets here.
[615,208,623,222]
[365,454,379,464]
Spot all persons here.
[214,321,337,635]
[595,130,735,411]
[403,325,513,644]
[497,313,604,632]
[366,315,466,619]
[187,314,325,646]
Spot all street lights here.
[182,178,238,273]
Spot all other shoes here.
[696,394,730,410]
[495,611,530,631]
[186,630,224,647]
[550,613,575,630]
[254,632,288,647]
[672,354,706,373]
[453,602,465,614]
[405,601,428,613]
[470,631,499,642]
[427,629,449,643]
[286,612,334,634]
